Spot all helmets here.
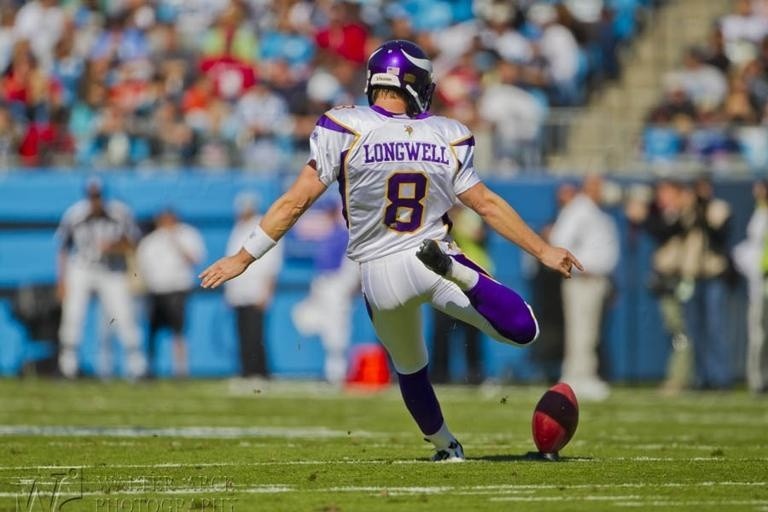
[364,39,436,115]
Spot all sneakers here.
[415,239,452,278]
[432,438,466,465]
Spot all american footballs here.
[532,383,578,452]
